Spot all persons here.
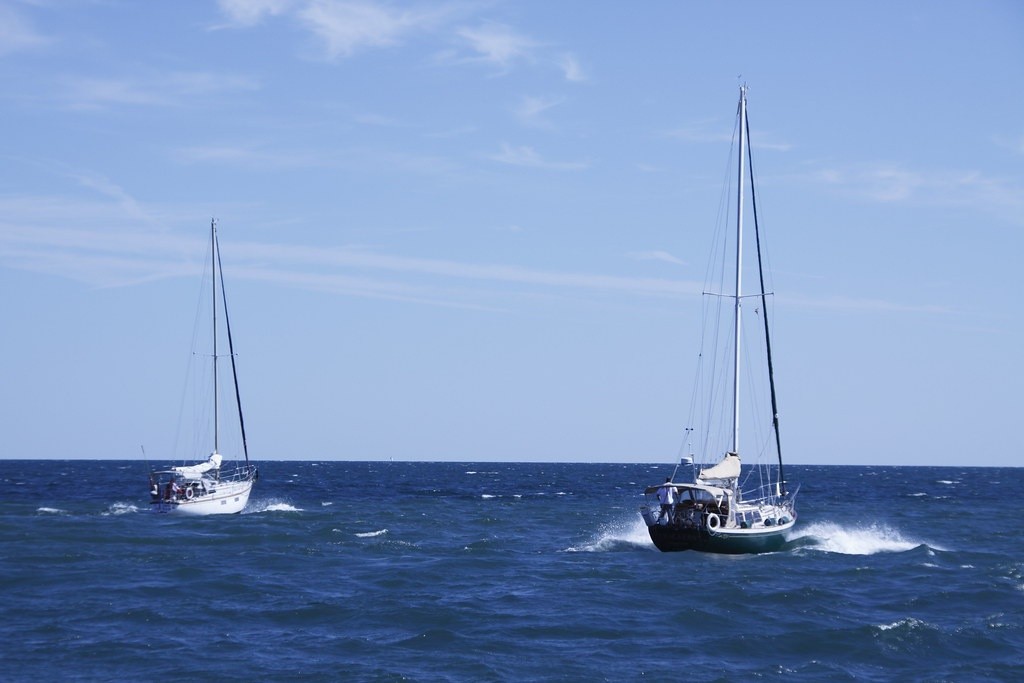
[167,480,182,499]
[656,477,679,525]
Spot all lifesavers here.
[707,513,720,531]
[185,487,194,500]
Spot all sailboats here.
[151,217,261,523]
[634,86,801,549]
